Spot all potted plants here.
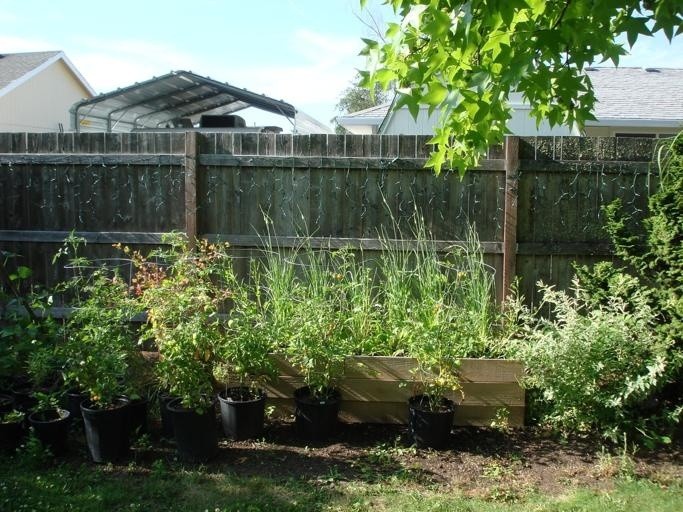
[1,224,465,464]
